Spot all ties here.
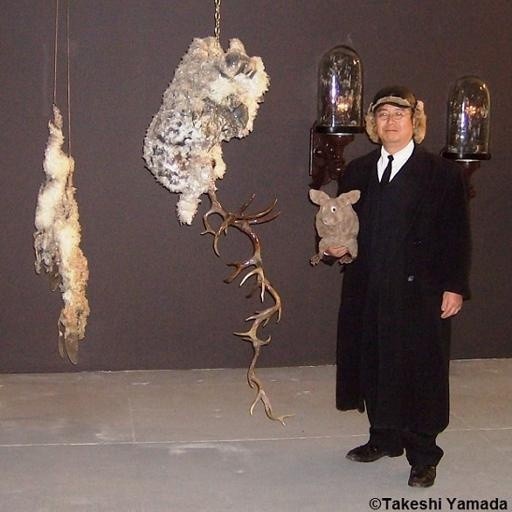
[379,155,394,183]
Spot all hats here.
[370,86,417,112]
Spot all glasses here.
[379,113,404,121]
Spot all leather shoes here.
[345,440,404,462]
[407,464,436,488]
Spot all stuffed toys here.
[308,187,362,267]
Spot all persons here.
[314,86,473,488]
